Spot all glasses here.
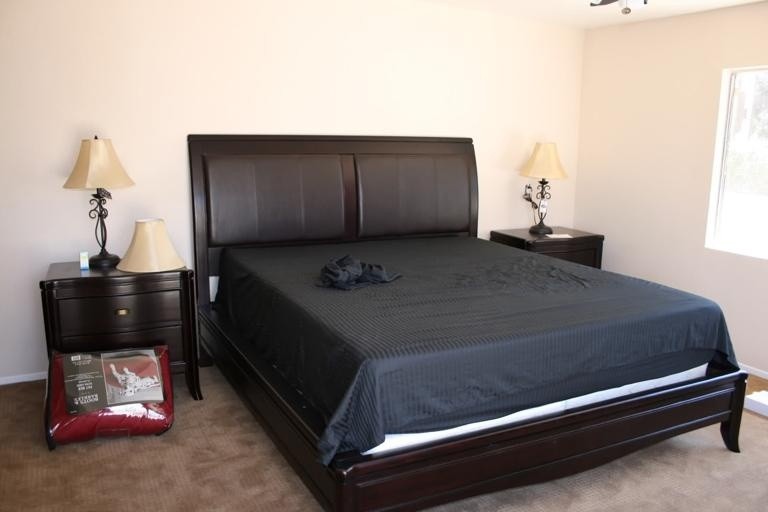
[62,134,136,271]
[518,141,568,235]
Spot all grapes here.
[39,258,204,400]
[490,226,604,269]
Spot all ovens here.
[186,133,751,511]
